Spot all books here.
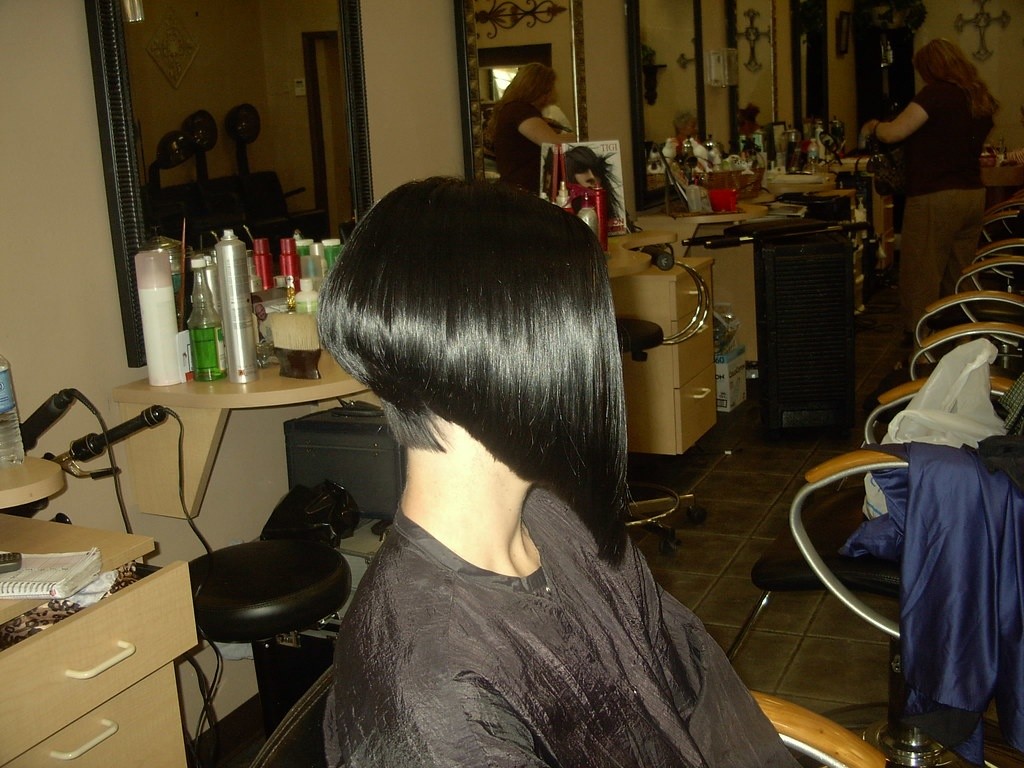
[0,546,104,601]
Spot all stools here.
[609,315,708,554]
[188,536,354,704]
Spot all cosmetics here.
[555,182,608,249]
[252,239,346,297]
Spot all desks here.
[607,228,678,279]
[112,346,370,524]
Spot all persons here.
[318,175,804,768]
[482,62,576,195]
[861,38,1002,376]
[672,111,704,155]
[543,146,618,229]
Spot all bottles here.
[677,135,720,212]
[784,115,846,169]
[0,353,25,464]
[536,182,609,258]
[134,229,347,386]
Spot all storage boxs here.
[714,344,748,413]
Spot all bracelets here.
[873,121,884,138]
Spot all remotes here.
[0,552,22,573]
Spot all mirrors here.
[450,1,590,220]
[84,1,379,370]
[726,1,783,167]
[625,0,708,215]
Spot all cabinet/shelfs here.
[870,177,894,262]
[635,203,767,256]
[850,231,866,316]
[1,514,201,768]
[618,256,720,459]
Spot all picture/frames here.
[768,119,786,157]
[837,10,850,53]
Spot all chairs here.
[752,187,1024,768]
